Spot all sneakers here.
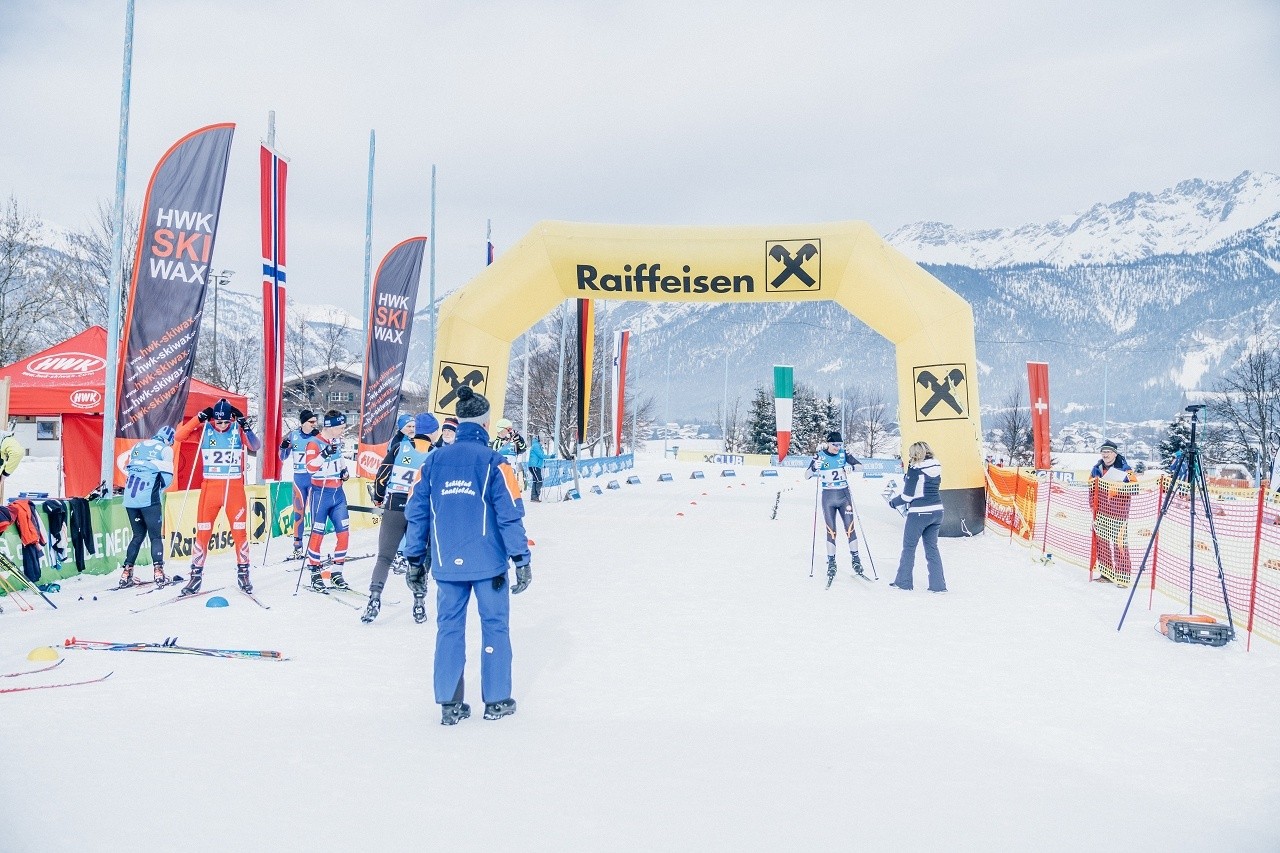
[361,598,381,622]
[852,560,863,572]
[413,600,427,623]
[119,547,350,593]
[827,561,839,576]
[390,556,406,573]
[441,702,471,725]
[484,699,515,719]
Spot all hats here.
[1100,440,1117,453]
[159,425,177,444]
[394,385,543,443]
[826,432,843,442]
[212,398,231,420]
[300,410,315,424]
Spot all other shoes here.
[928,588,948,594]
[1118,583,1127,588]
[890,583,900,588]
[1093,577,1113,583]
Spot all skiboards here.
[253,553,376,574]
[825,572,875,591]
[105,578,188,597]
[0,553,57,610]
[128,584,272,614]
[303,586,402,611]
[64,635,291,662]
[1,657,116,693]
[345,485,385,516]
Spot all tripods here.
[1118,415,1234,631]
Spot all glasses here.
[831,443,841,447]
[217,422,229,425]
[308,420,316,424]
[1102,451,1114,455]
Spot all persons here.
[987,456,1003,467]
[0,430,25,480]
[367,412,460,622]
[173,400,261,595]
[486,418,558,504]
[1087,441,1139,588]
[403,385,532,726]
[117,426,175,586]
[890,442,947,593]
[804,432,865,575]
[276,409,351,591]
[1168,450,1188,498]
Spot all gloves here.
[231,406,252,432]
[509,564,531,595]
[406,564,427,594]
[198,406,214,422]
[281,439,349,481]
[889,501,896,510]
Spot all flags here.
[1027,362,1050,469]
[257,144,287,479]
[612,332,629,457]
[773,366,793,462]
[357,236,428,481]
[112,122,235,439]
[577,298,594,443]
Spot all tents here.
[0,325,247,498]
